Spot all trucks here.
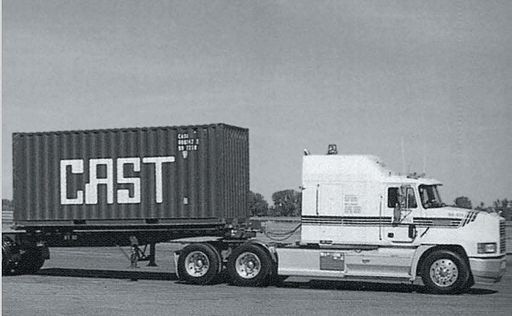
[1,123,506,295]
